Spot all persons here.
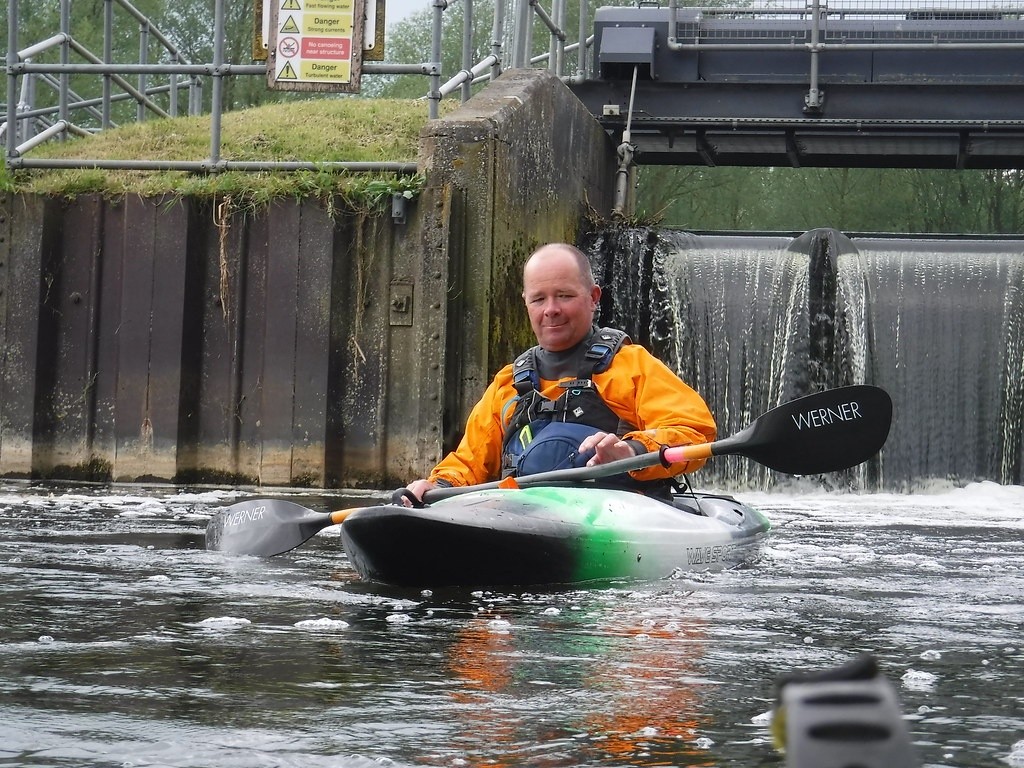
[402,243,717,501]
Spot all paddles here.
[206,379,898,566]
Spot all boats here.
[340,486,770,590]
